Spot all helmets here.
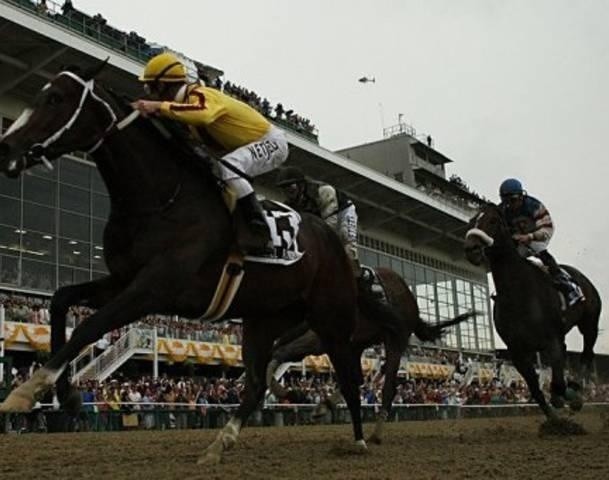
[139,53,187,84]
[499,179,523,196]
[276,167,305,186]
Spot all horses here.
[462,197,604,429]
[0,56,406,471]
[249,264,486,445]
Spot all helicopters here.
[358,75,376,85]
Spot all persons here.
[449,174,490,207]
[276,166,384,294]
[427,135,432,147]
[131,51,290,257]
[496,178,583,306]
[36,0,316,135]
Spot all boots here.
[230,193,274,254]
[555,269,587,306]
